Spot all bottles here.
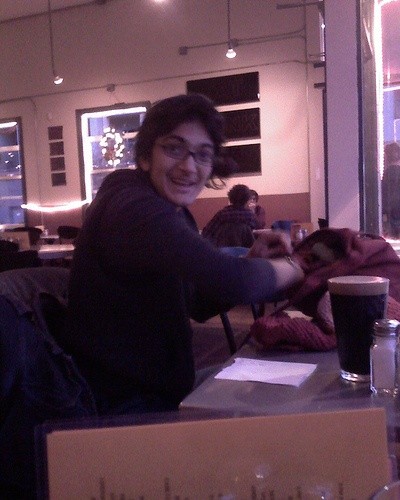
[369,318,400,398]
[295,229,303,240]
[304,229,308,239]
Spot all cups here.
[328,276,390,382]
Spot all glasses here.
[154,140,218,168]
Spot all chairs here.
[57,225,80,242]
[0,266,98,419]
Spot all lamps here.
[100,126,124,167]
[47,0,63,85]
[226,0,237,58]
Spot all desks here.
[38,243,75,266]
[180,332,400,443]
[40,234,59,244]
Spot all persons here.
[202,184,258,247]
[382,143,400,236]
[68,95,306,415]
[249,189,265,230]
